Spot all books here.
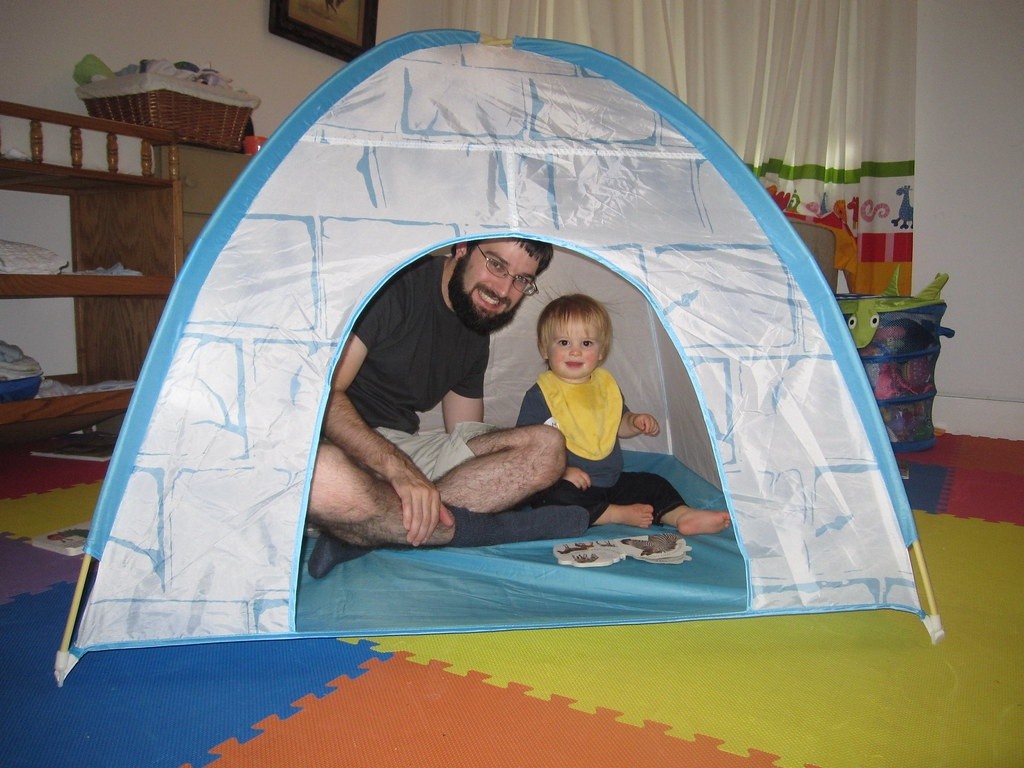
[32,519,92,557]
[30,432,120,462]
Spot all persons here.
[306,235,592,580]
[515,293,731,537]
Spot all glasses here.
[477,243,539,296]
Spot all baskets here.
[76,72,261,152]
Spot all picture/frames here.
[268,0,379,62]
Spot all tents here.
[50,32,945,685]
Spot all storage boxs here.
[76,73,261,153]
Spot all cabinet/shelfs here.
[0,101,186,450]
[153,144,252,265]
